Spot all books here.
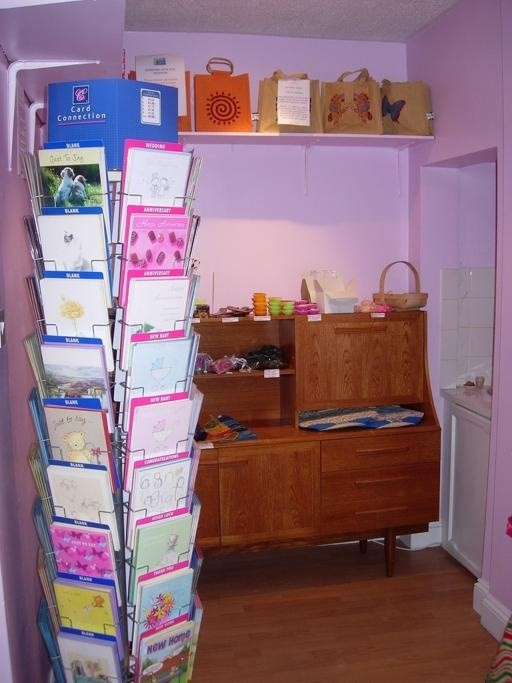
[29,80,202,681]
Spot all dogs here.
[69,174,87,205]
[52,166,75,208]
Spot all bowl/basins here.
[251,293,320,316]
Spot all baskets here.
[373,262,428,309]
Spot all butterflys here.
[382,95,406,122]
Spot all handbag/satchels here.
[321,68,384,135]
[380,79,434,136]
[256,69,325,133]
[194,58,253,133]
